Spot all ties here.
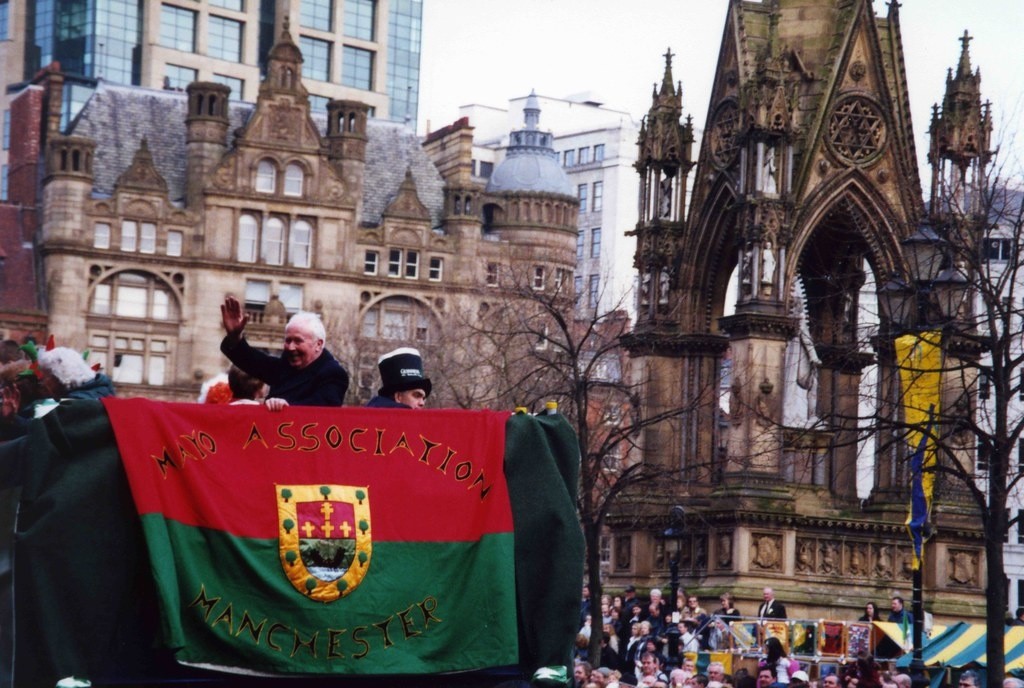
[763,603,769,617]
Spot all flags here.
[100,397,520,678]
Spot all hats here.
[625,586,635,592]
[597,667,611,677]
[620,672,637,688]
[792,670,809,681]
[378,347,432,398]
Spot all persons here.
[219,294,349,412]
[367,347,432,410]
[570,582,1023,688]
[0,337,118,439]
